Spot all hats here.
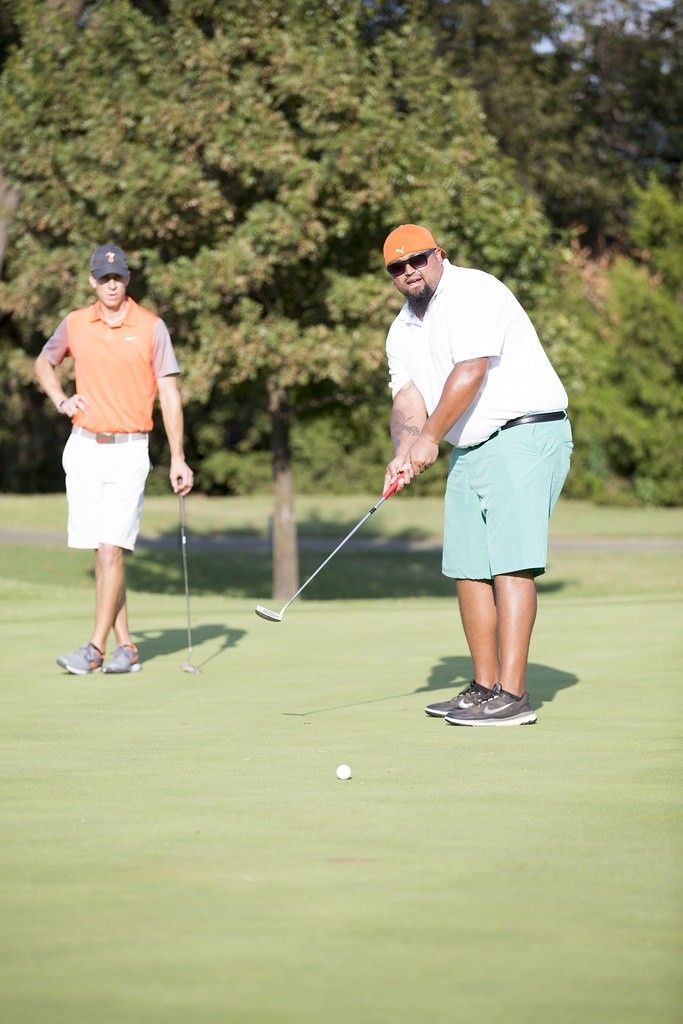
[382,223,447,265]
[92,244,129,278]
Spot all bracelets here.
[56,400,66,410]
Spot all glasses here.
[386,249,435,278]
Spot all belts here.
[493,410,568,436]
[75,426,147,444]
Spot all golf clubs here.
[178,476,199,673]
[255,471,405,622]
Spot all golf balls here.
[337,765,351,780]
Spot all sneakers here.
[424,682,492,717]
[57,643,104,675]
[102,644,143,674]
[445,683,539,728]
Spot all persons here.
[32,245,194,676]
[382,226,572,729]
